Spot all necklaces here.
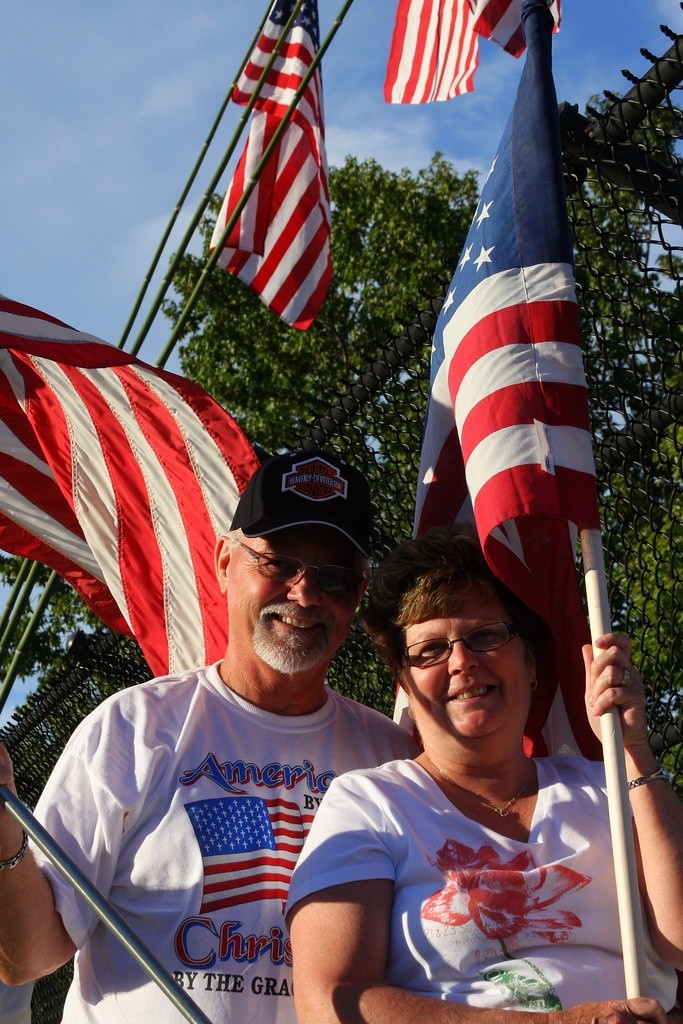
[421,752,537,818]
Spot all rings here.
[621,666,630,686]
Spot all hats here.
[231,449,375,558]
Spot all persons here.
[0,449,422,1024]
[283,529,681,1024]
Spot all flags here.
[210,1,332,332]
[383,0,482,106]
[472,0,563,60]
[388,9,619,767]
[0,291,288,689]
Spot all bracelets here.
[622,767,670,792]
[1,828,30,874]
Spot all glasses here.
[231,538,368,595]
[401,620,518,671]
